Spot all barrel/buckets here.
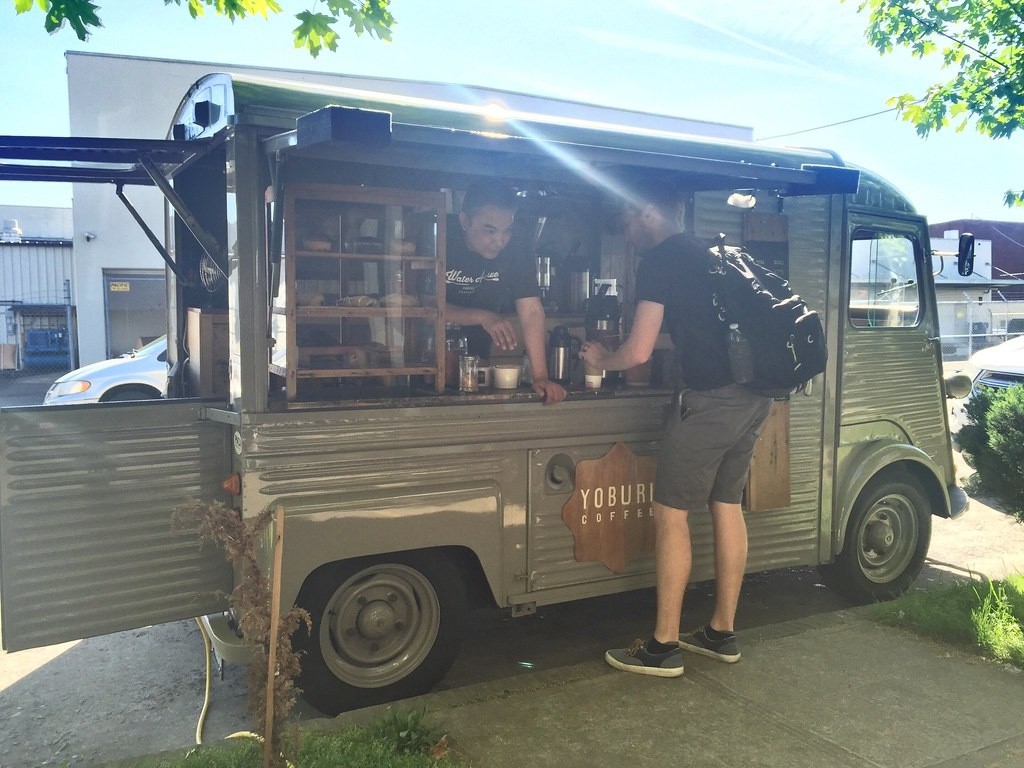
[585,282,622,386]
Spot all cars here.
[965,332,1023,392]
[42,295,276,406]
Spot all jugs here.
[548,326,584,384]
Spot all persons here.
[578,179,774,679]
[416,180,567,406]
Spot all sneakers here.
[679,624,742,663]
[605,638,684,678]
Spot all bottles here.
[422,322,469,388]
[726,323,755,385]
[458,352,478,394]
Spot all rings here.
[583,345,588,351]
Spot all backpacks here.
[676,234,829,403]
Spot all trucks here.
[0,71,974,721]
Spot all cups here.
[476,366,520,389]
[585,375,601,388]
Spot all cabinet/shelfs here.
[262,181,448,395]
